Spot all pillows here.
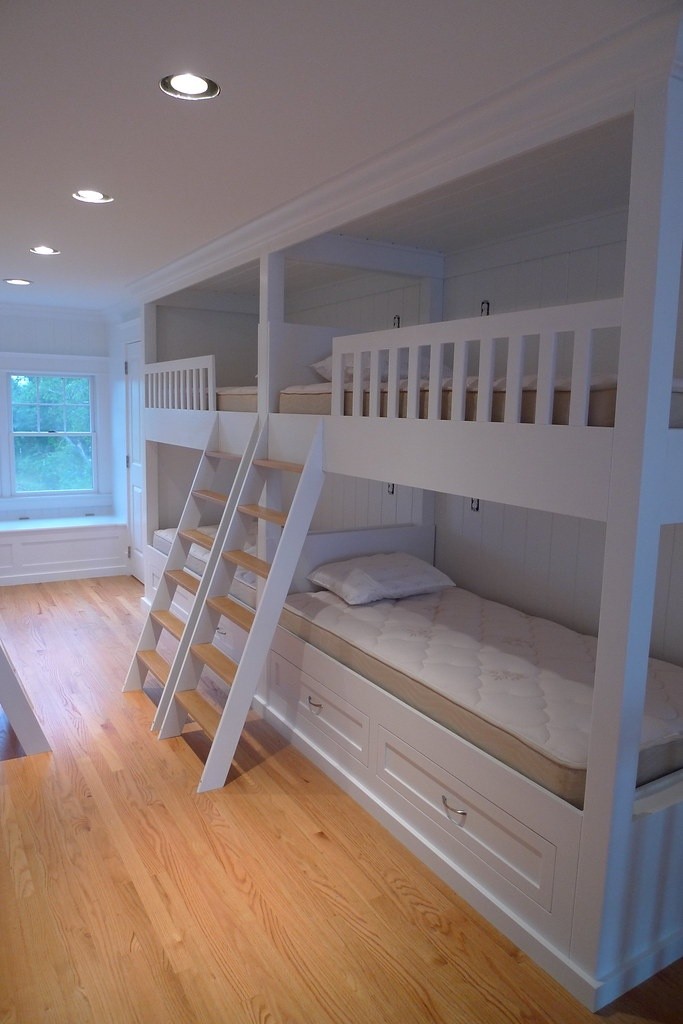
[305,551,458,607]
[311,346,454,384]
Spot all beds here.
[119,0,683,1011]
